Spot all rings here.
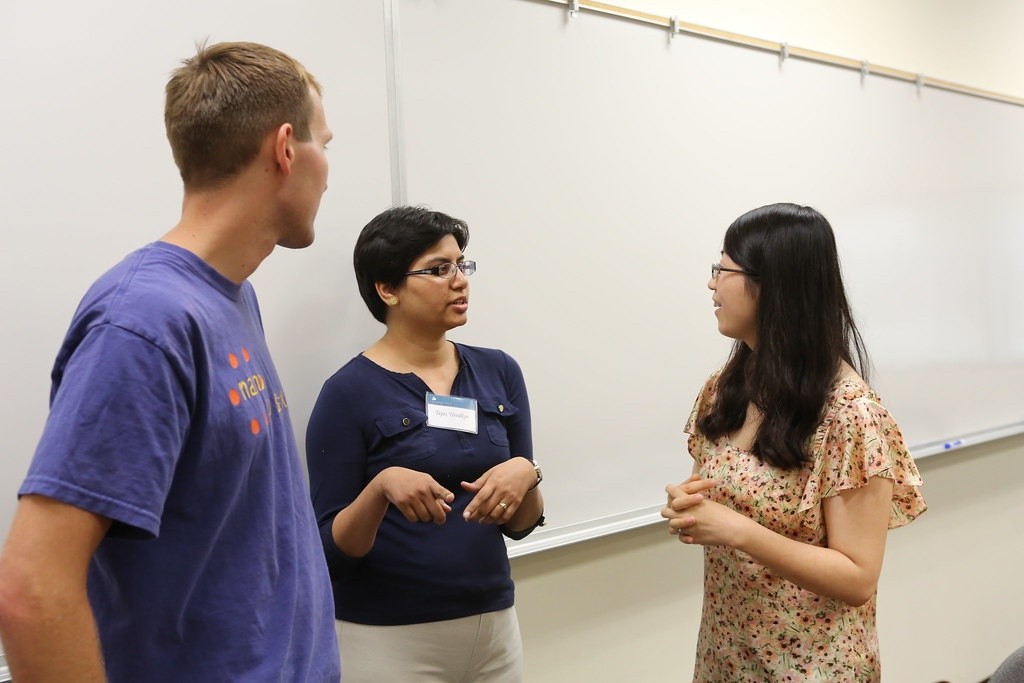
[499,503,507,510]
[679,528,683,535]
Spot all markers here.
[944,440,964,448]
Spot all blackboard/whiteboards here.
[380,0,1024,558]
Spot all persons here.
[306,207,544,683]
[661,203,926,683]
[0,42,340,683]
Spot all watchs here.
[528,459,542,492]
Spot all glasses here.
[397,261,476,278]
[712,264,759,279]
